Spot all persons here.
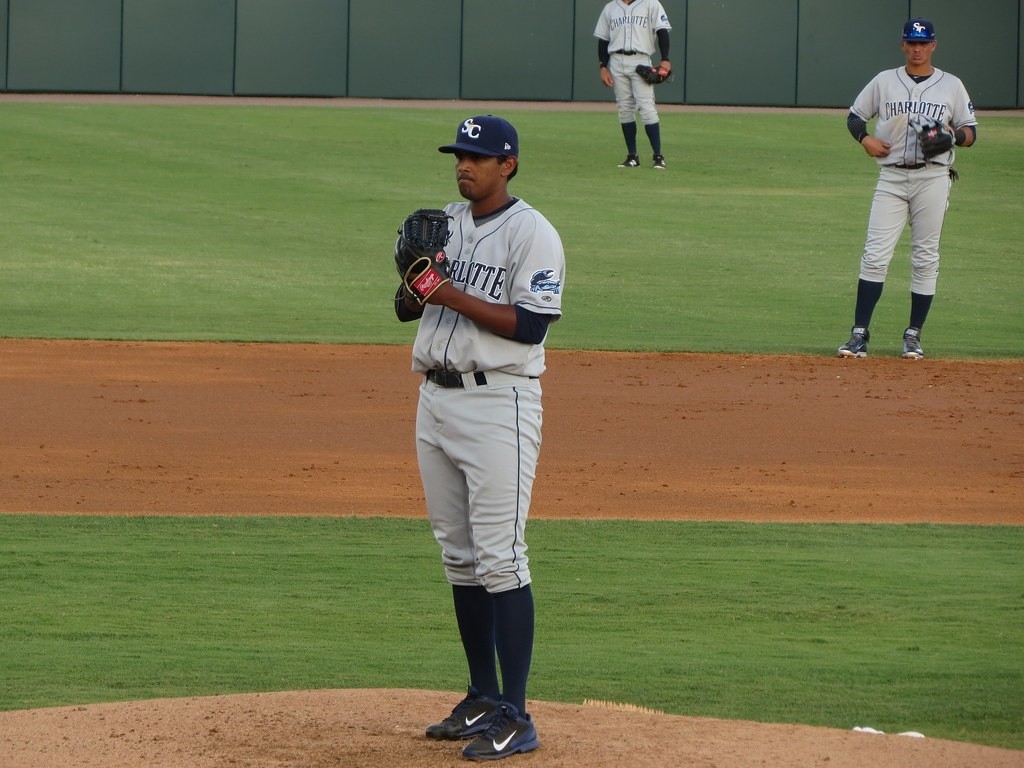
[395,115,567,759]
[593,0,671,169]
[839,18,979,359]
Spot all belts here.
[616,49,643,56]
[895,162,938,169]
[426,368,539,388]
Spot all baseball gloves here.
[635,64,672,85]
[908,113,957,161]
[395,208,455,307]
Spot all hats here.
[439,115,518,158]
[902,17,935,42]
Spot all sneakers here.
[425,684,504,740]
[618,154,640,167]
[462,701,538,759]
[902,327,923,358]
[654,154,666,169]
[838,326,869,357]
